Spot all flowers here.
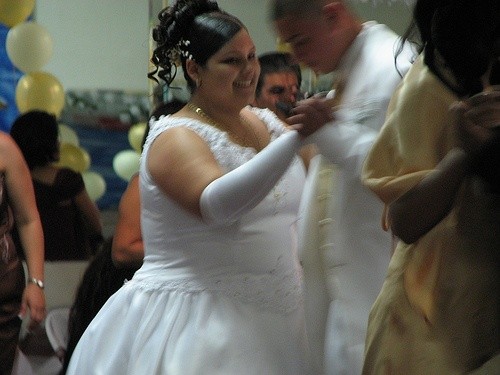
[163,40,196,68]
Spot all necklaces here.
[186,101,261,152]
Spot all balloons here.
[0,0,150,205]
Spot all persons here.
[360,0,500,375]
[449,101,500,185]
[66,0,334,375]
[0,52,302,375]
[267,0,419,375]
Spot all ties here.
[333,76,346,99]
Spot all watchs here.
[27,277,45,289]
